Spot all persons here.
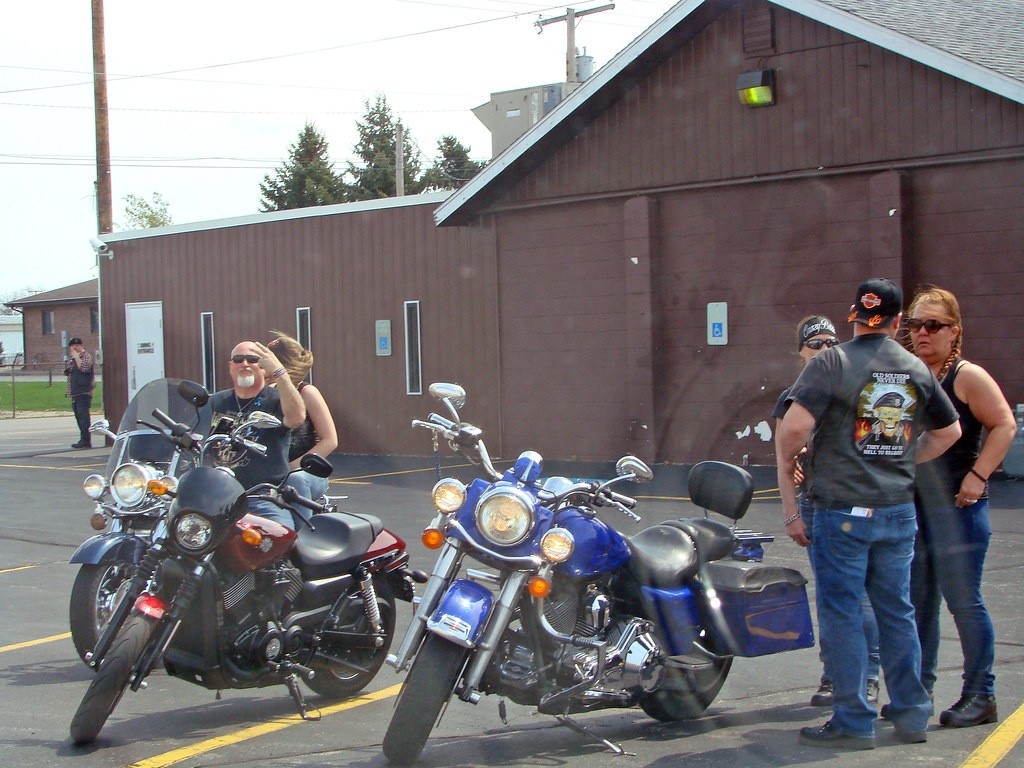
[774,278,1018,749]
[64,339,95,448]
[187,331,338,533]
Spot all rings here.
[963,498,977,504]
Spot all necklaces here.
[235,391,261,417]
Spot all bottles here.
[1002,404,1024,475]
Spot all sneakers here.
[881,689,936,719]
[798,718,877,750]
[939,694,998,727]
[865,679,880,704]
[810,681,834,705]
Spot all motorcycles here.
[69,377,348,665]
[70,381,427,744]
[382,382,816,767]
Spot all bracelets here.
[270,366,287,380]
[783,513,800,527]
[970,469,986,483]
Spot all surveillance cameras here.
[89,237,108,252]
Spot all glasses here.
[228,354,260,363]
[802,338,839,350]
[905,318,952,334]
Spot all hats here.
[845,277,904,328]
[68,338,83,345]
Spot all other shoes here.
[71,437,92,448]
[895,727,929,743]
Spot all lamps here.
[736,67,778,110]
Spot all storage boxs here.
[693,559,815,658]
